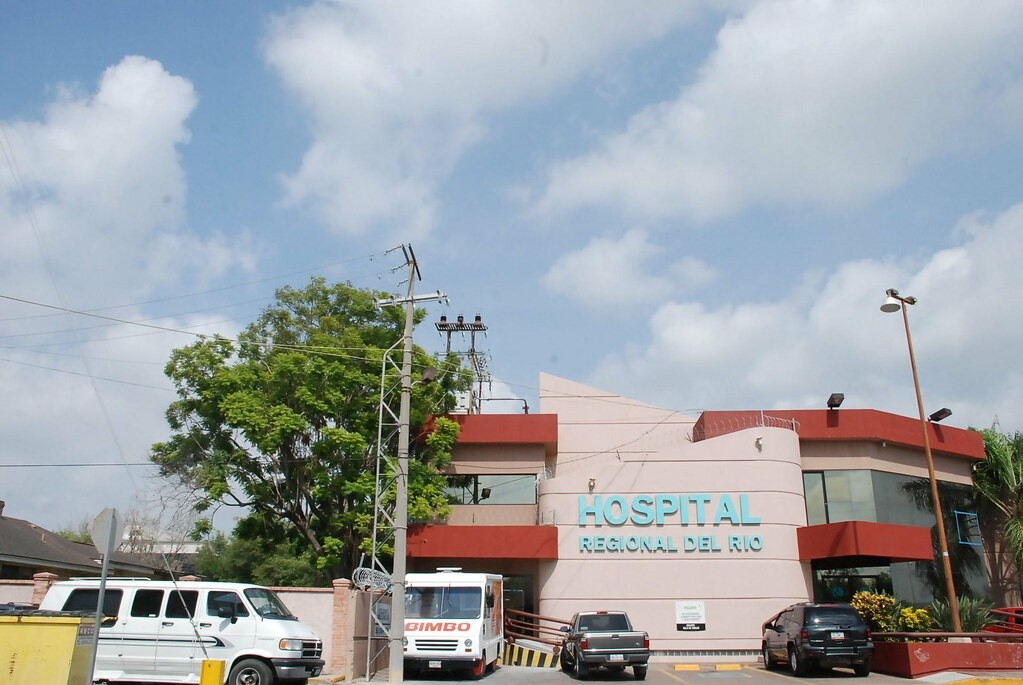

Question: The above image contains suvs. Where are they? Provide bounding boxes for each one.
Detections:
[761,601,874,677]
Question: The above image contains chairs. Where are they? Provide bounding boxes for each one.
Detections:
[583,619,593,624]
[609,617,621,624]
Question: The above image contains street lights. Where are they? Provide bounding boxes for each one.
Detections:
[879,288,966,643]
[371,289,451,684]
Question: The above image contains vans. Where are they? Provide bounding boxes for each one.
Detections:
[37,576,326,685]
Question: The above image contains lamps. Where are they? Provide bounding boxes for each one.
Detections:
[928,407,952,422]
[827,393,845,409]
[757,436,763,444]
[588,478,595,489]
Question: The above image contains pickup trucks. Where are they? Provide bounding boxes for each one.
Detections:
[560,610,650,681]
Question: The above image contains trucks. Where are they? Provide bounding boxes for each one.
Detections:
[401,567,504,677]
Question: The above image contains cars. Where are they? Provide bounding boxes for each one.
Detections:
[980,606,1023,634]
